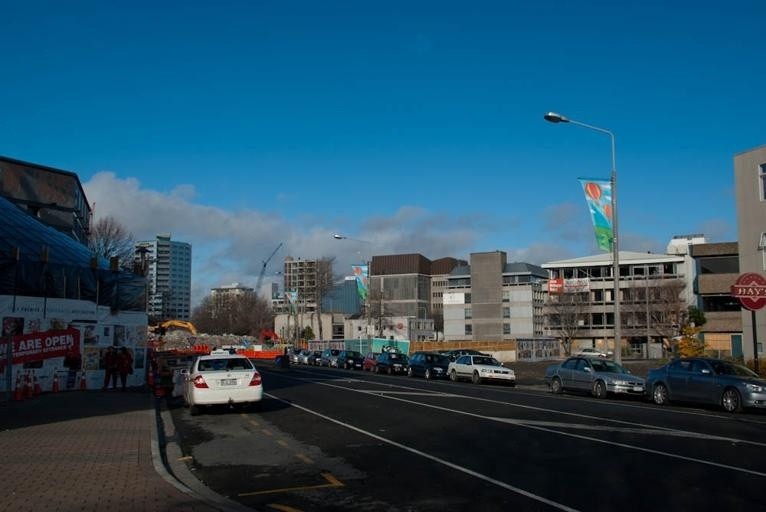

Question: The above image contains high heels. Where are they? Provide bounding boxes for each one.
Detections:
[147,365,154,387]
[51,367,60,393]
[78,368,88,390]
[13,367,42,402]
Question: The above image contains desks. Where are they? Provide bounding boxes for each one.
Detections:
[334,234,372,340]
[543,111,624,368]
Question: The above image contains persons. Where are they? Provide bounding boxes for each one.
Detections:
[115,347,133,388]
[100,346,117,391]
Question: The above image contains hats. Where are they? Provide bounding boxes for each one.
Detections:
[643,355,765,417]
[181,353,264,417]
[544,356,646,402]
[573,347,607,359]
[286,346,518,385]
